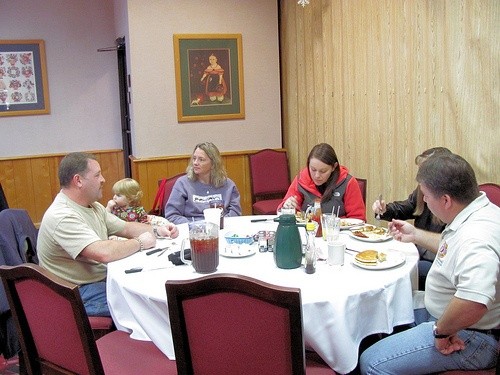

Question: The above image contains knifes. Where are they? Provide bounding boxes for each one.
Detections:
[146,246,169,256]
[251,218,275,222]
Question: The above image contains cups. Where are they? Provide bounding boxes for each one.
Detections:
[281,206,295,215]
[328,241,345,267]
[209,200,225,229]
[320,212,340,241]
[203,208,221,228]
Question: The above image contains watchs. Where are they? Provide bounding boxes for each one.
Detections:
[432,322,449,339]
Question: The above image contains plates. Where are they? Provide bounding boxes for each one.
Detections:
[353,249,406,270]
[296,220,305,226]
[219,244,256,258]
[340,218,393,243]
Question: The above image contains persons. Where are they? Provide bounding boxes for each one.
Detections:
[372,147,452,289]
[37,151,179,316]
[360,153,500,375]
[277,143,367,223]
[165,142,241,225]
[106,178,150,225]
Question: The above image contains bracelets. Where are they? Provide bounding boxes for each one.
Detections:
[153,225,160,236]
[135,238,143,251]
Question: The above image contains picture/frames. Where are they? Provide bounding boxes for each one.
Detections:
[172,34,246,122]
[0,38,52,117]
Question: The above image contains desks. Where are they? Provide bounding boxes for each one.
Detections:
[105,216,419,375]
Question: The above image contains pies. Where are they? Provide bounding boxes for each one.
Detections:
[355,250,379,266]
[365,225,374,231]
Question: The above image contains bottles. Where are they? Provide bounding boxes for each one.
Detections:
[267,230,276,253]
[304,223,316,274]
[257,230,267,253]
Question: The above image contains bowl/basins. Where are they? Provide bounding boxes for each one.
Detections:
[224,230,255,245]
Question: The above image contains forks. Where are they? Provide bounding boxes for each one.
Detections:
[376,193,383,220]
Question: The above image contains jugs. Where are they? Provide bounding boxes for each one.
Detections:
[179,220,219,274]
[306,206,322,237]
[274,213,308,269]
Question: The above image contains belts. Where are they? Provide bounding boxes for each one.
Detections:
[464,327,500,336]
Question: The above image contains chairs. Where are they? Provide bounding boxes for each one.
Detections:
[248,148,291,216]
[0,263,178,375]
[163,274,336,375]
[0,210,112,375]
